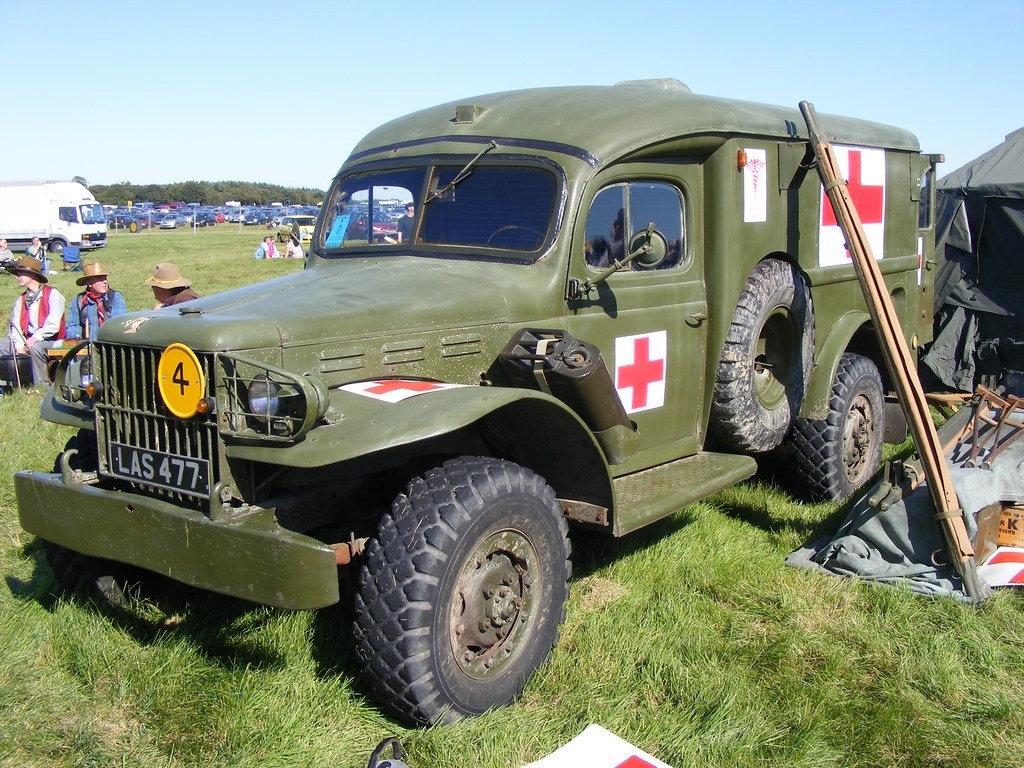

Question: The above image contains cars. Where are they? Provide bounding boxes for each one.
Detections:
[90,201,413,241]
[276,215,330,245]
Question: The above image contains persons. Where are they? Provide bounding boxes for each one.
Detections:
[0,202,416,388]
[582,211,681,273]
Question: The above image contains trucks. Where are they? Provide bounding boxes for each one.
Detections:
[1,180,108,255]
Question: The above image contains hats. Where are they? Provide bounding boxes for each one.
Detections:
[142,263,193,289]
[75,262,112,286]
[6,256,49,284]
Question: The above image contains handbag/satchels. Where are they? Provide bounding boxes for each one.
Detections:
[254,246,265,259]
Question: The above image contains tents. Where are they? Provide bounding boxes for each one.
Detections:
[918,127,1024,397]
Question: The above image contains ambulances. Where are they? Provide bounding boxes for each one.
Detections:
[12,76,947,728]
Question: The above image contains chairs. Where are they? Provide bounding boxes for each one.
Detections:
[60,246,88,272]
[26,247,50,274]
